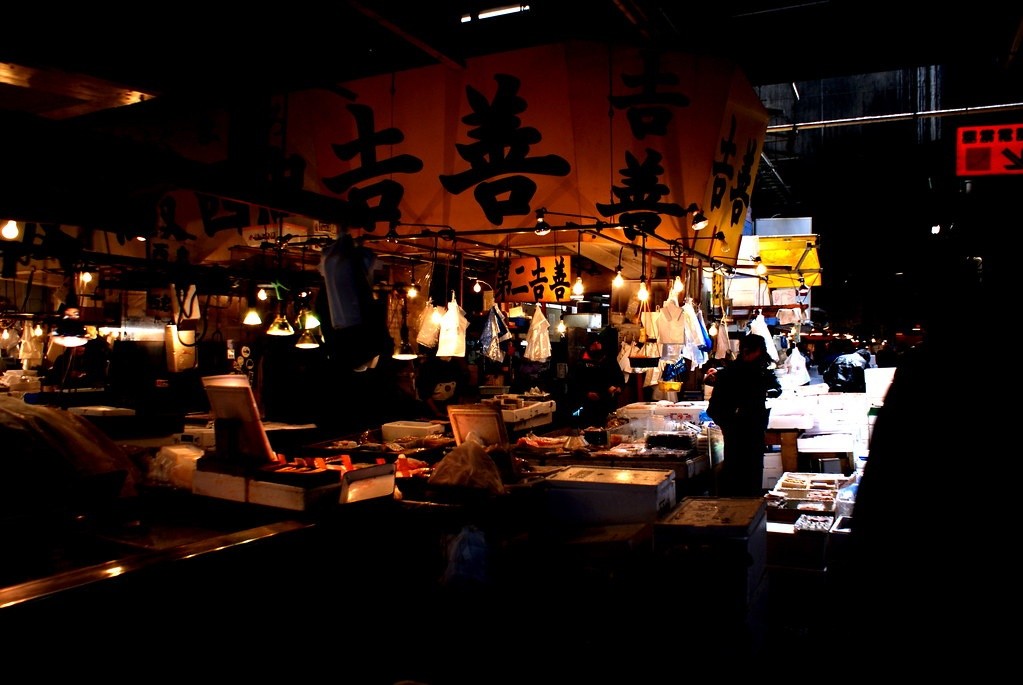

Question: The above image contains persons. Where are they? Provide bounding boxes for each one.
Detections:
[822,340,909,392]
[577,339,626,428]
[0,296,587,436]
[852,175,1023,684]
[706,333,781,497]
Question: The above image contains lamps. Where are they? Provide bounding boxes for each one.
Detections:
[536,209,551,236]
[392,305,418,360]
[385,222,399,243]
[688,203,709,230]
[50,318,89,348]
[244,288,322,349]
[753,256,767,275]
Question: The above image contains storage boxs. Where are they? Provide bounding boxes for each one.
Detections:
[762,367,897,489]
[546,464,677,525]
[192,461,396,511]
[615,399,709,421]
[381,420,444,440]
